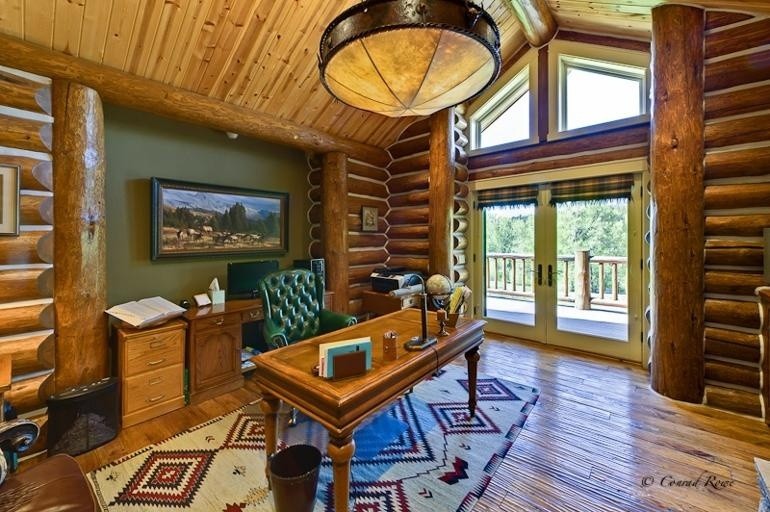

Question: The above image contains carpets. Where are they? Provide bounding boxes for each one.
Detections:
[86,363,541,511]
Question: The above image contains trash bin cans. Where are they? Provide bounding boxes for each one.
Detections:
[268,444,322,512]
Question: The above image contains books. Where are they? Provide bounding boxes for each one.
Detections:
[317,335,373,380]
[104,296,186,329]
[240,346,263,369]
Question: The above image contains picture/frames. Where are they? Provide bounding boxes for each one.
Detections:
[0,164,20,236]
[361,205,379,232]
[150,176,289,262]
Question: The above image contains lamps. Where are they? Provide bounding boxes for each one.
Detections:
[390,274,438,352]
[317,0,501,118]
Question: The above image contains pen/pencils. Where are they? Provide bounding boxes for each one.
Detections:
[384,330,399,339]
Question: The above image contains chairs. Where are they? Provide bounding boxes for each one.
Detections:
[258,268,357,351]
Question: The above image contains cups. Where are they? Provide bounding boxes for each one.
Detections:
[382,336,398,361]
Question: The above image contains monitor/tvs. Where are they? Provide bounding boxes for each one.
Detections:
[226,259,280,302]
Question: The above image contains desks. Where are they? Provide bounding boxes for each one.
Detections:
[250,307,489,512]
[362,290,421,321]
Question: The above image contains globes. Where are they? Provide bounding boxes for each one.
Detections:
[426,273,454,336]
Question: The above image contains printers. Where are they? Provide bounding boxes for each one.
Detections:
[370,270,424,292]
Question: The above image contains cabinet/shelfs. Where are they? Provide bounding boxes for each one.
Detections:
[112,318,189,430]
[182,298,264,407]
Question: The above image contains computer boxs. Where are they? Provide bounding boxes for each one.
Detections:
[293,258,326,294]
